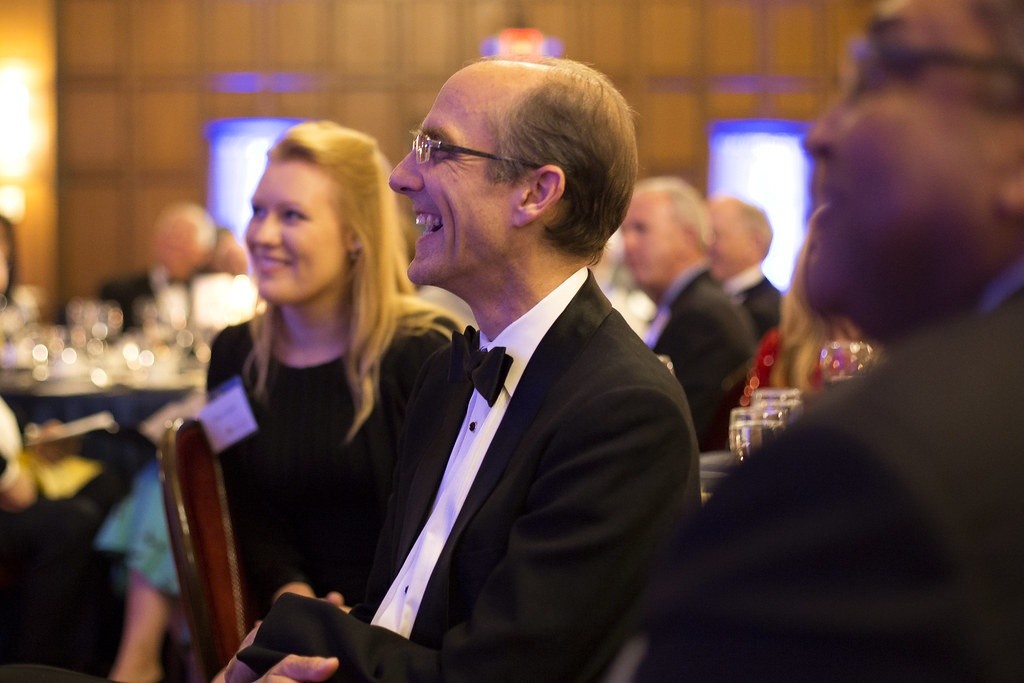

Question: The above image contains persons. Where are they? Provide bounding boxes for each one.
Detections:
[112,123,460,682]
[620,177,782,454]
[101,206,214,335]
[618,1,1022,683]
[0,398,80,513]
[94,465,183,683]
[217,61,697,682]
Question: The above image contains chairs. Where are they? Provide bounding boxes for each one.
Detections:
[156,417,256,683]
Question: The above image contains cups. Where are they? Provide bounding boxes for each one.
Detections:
[728,387,804,470]
[820,340,873,384]
[0,271,259,386]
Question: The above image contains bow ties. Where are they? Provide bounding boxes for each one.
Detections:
[449,325,508,407]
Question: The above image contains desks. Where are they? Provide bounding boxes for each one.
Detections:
[0,370,199,485]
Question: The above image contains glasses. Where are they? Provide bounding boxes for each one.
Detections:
[411,130,571,202]
[850,33,1024,95]
